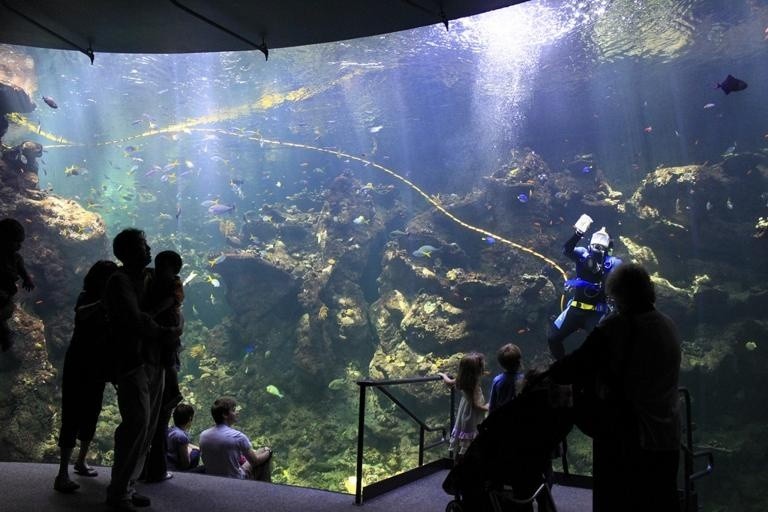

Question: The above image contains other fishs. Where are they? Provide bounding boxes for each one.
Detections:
[0,112,350,360]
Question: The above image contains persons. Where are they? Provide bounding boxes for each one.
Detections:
[199,398,273,482]
[529,265,681,511]
[166,404,206,473]
[0,217,184,511]
[548,213,623,360]
[2,218,33,353]
[450,343,525,463]
[52,228,182,512]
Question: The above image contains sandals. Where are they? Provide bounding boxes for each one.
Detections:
[74,461,98,477]
[56,476,79,490]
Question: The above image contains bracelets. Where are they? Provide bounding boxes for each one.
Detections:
[264,449,273,458]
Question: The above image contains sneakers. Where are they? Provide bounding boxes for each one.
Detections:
[106,491,150,512]
